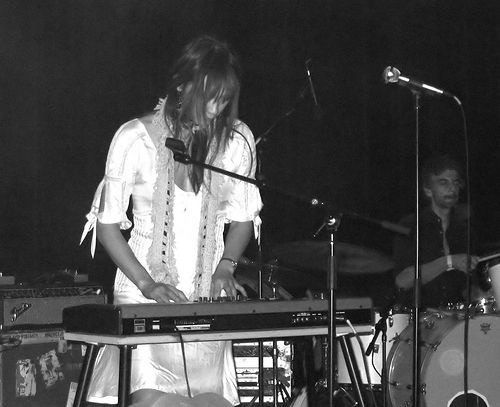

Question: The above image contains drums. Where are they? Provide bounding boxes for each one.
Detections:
[382,305,499,407]
[461,251,500,315]
[310,305,413,388]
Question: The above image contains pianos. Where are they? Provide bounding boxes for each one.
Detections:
[61,296,374,347]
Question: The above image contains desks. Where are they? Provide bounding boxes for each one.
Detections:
[63,324,374,407]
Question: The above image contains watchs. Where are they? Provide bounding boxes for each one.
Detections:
[446,255,454,272]
[219,258,238,268]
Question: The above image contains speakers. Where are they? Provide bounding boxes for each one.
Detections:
[0,328,86,407]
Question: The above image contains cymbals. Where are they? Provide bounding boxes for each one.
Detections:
[265,238,398,276]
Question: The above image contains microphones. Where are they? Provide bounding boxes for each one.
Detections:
[307,69,317,105]
[383,66,454,99]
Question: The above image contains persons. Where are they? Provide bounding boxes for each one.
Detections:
[392,157,485,311]
[79,37,263,407]
